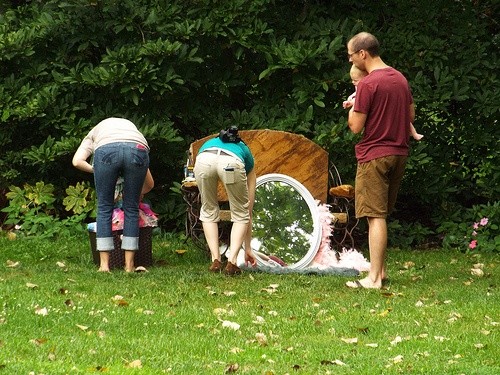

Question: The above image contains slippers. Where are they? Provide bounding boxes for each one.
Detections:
[346,280,363,289]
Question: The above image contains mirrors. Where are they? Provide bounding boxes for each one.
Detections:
[245,173,323,267]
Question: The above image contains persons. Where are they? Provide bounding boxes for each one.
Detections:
[345,32,415,291]
[342,64,424,140]
[71,117,154,272]
[194,136,257,273]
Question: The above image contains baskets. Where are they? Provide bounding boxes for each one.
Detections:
[88,226,153,267]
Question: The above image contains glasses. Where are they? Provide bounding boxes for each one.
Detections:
[348,48,366,59]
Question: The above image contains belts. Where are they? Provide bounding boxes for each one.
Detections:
[203,150,233,157]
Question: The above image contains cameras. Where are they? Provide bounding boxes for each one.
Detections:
[219,125,242,143]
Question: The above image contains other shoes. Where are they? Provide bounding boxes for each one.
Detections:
[225,262,241,274]
[210,260,226,272]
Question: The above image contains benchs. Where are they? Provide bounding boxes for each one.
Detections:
[181,124,360,252]
